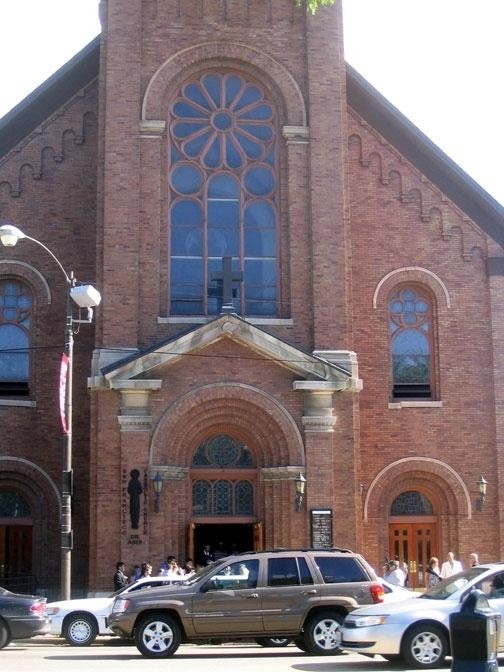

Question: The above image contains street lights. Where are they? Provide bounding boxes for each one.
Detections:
[0,222,76,601]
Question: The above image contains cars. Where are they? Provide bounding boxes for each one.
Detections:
[47,575,292,648]
[0,586,52,648]
[337,560,504,669]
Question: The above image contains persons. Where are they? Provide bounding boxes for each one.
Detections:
[114,542,257,592]
[488,578,504,598]
[381,560,408,588]
[440,551,464,593]
[423,556,442,590]
[469,553,482,567]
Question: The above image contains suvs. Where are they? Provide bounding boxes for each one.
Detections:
[109,547,384,658]
